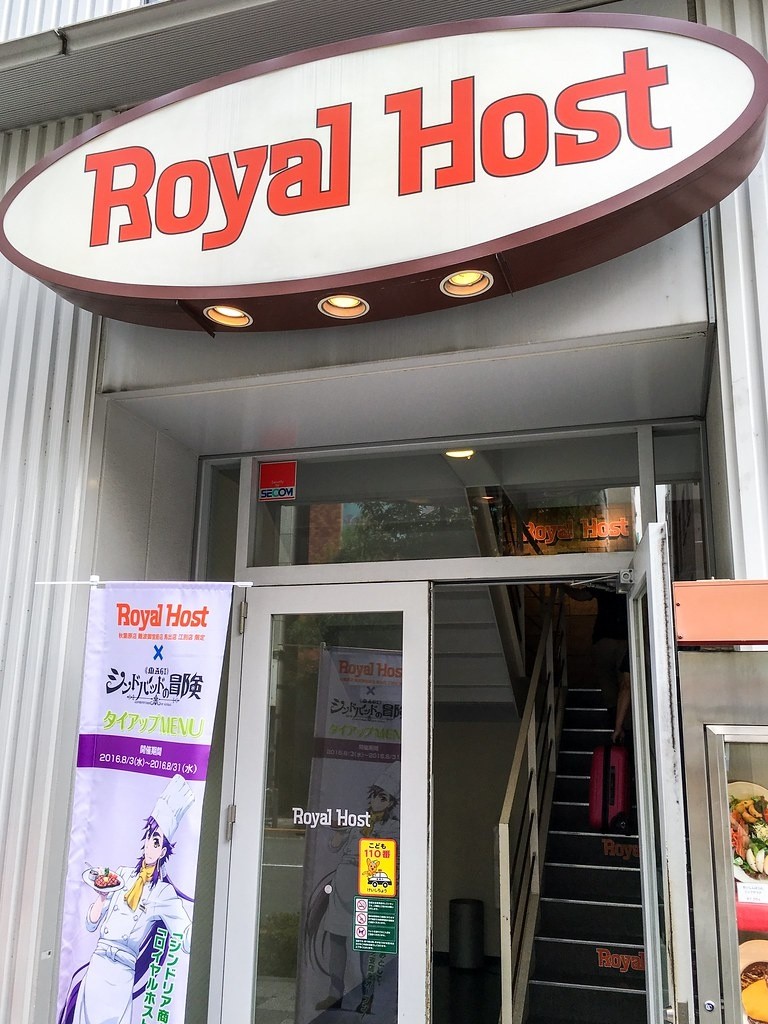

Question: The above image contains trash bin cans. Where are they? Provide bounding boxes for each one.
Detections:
[449,898,484,970]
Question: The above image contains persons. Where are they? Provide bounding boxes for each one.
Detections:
[550,582,637,836]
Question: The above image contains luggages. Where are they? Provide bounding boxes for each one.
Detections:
[589,735,631,834]
